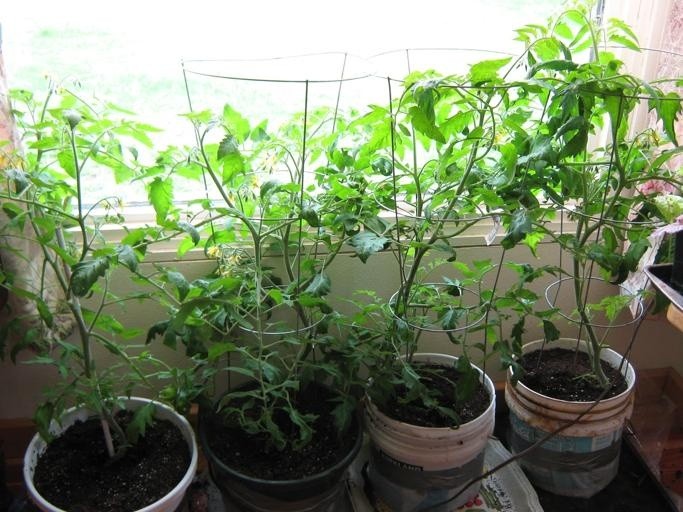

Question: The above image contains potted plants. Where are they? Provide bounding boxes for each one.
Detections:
[491,2,683,501]
[167,67,369,512]
[2,73,204,512]
[353,55,534,511]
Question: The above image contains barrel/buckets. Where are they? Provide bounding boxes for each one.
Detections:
[363,352,496,512]
[24,396,201,512]
[505,338,636,499]
[198,376,363,512]
